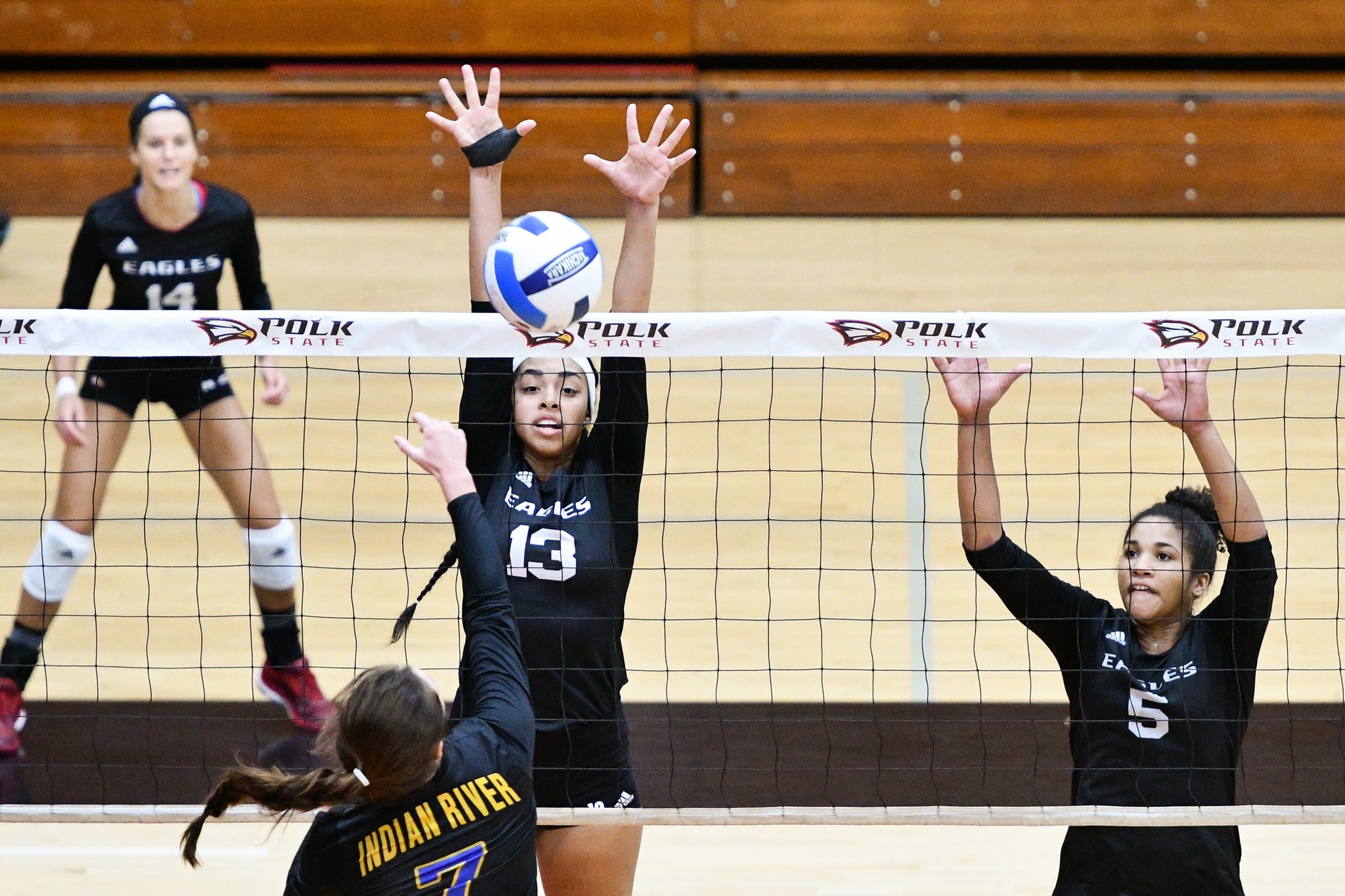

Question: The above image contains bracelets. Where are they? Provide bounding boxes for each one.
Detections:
[55,375,78,398]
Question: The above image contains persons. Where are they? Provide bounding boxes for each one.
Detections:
[391,61,695,896]
[0,86,334,754]
[932,359,1278,896]
[175,413,539,896]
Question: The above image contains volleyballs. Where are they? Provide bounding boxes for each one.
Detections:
[483,210,604,334]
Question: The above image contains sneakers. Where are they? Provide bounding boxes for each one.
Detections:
[0,677,27,755]
[254,656,329,731]
